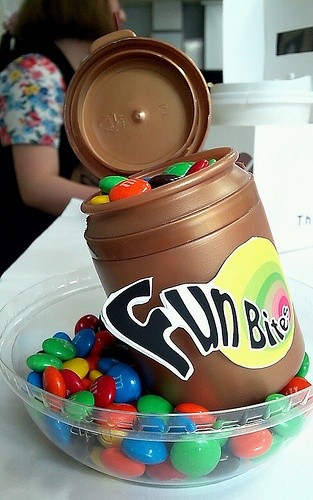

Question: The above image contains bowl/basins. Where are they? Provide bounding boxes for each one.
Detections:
[0,266,313,487]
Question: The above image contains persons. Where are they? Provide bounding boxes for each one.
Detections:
[0,0,127,277]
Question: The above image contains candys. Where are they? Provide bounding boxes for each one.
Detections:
[85,158,219,207]
[25,314,313,481]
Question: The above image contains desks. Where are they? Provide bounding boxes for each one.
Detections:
[0,193,313,500]
